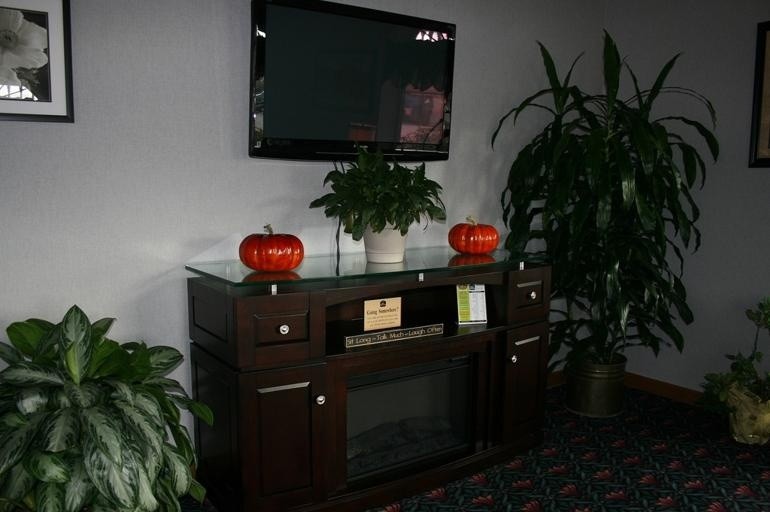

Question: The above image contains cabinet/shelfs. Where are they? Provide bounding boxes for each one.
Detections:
[182,237,564,509]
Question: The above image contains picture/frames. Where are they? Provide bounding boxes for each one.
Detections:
[1,0,77,124]
[746,19,769,171]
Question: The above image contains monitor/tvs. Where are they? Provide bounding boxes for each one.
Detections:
[249,0,456,162]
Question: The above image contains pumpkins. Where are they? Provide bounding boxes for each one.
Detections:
[448,224,499,254]
[239,234,304,272]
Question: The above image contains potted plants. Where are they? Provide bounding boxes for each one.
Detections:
[486,24,723,425]
[304,134,449,277]
[695,293,769,449]
